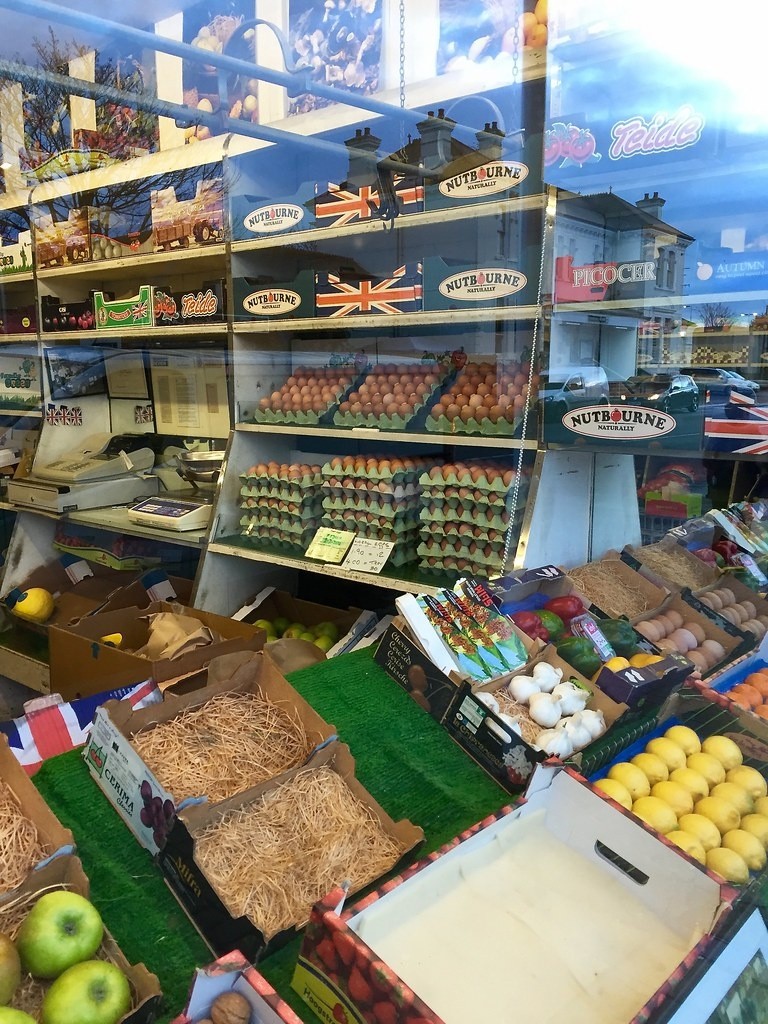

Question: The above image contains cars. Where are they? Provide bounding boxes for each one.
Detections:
[621,373,698,415]
[727,370,759,392]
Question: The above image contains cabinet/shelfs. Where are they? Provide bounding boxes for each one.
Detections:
[0,38,768,621]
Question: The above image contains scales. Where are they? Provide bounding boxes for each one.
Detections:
[127,450,226,533]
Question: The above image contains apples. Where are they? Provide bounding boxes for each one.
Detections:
[0,891,130,1024]
[253,617,341,653]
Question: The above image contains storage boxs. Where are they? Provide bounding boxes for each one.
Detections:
[0,521,201,635]
[0,651,426,1024]
[232,586,396,659]
[232,244,542,323]
[48,601,267,702]
[290,755,749,1024]
[0,426,39,480]
[0,179,225,274]
[232,132,543,242]
[638,491,703,546]
[0,278,227,334]
[0,345,43,411]
[373,498,767,909]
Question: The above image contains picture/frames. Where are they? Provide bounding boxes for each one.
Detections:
[43,345,108,400]
[648,904,768,1024]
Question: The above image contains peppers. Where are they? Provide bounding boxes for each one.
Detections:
[690,541,740,570]
[514,595,662,683]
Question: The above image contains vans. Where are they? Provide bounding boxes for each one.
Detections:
[679,366,757,401]
[531,366,610,416]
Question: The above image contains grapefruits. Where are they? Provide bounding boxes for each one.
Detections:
[15,588,54,621]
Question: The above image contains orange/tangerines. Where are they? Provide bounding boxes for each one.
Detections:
[722,667,768,722]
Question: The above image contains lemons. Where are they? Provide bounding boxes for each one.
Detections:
[592,725,768,884]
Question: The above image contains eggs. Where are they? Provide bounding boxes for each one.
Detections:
[634,587,768,673]
[238,363,543,577]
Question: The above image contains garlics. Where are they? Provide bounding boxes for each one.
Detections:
[474,663,605,758]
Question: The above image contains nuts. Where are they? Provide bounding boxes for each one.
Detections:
[195,992,252,1024]
